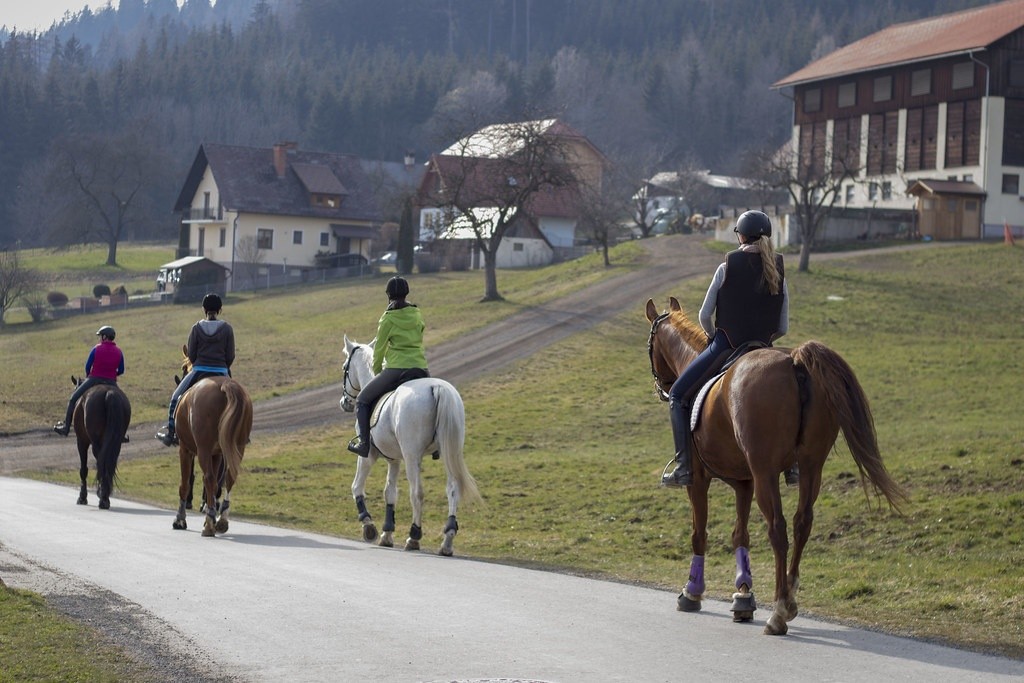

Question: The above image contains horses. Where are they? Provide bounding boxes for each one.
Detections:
[173,344,253,537]
[71,374,131,510]
[339,333,488,556]
[645,296,912,636]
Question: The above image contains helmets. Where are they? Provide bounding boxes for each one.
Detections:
[737,210,771,236]
[385,276,409,298]
[96,326,115,338]
[202,294,222,311]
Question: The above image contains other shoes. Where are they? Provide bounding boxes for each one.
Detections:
[155,432,173,446]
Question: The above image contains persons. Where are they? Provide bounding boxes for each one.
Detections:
[663,210,799,487]
[54,326,129,443]
[157,293,250,447]
[347,275,440,460]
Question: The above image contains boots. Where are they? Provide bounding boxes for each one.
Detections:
[662,397,692,484]
[348,400,373,458]
[784,464,799,486]
[54,403,75,436]
